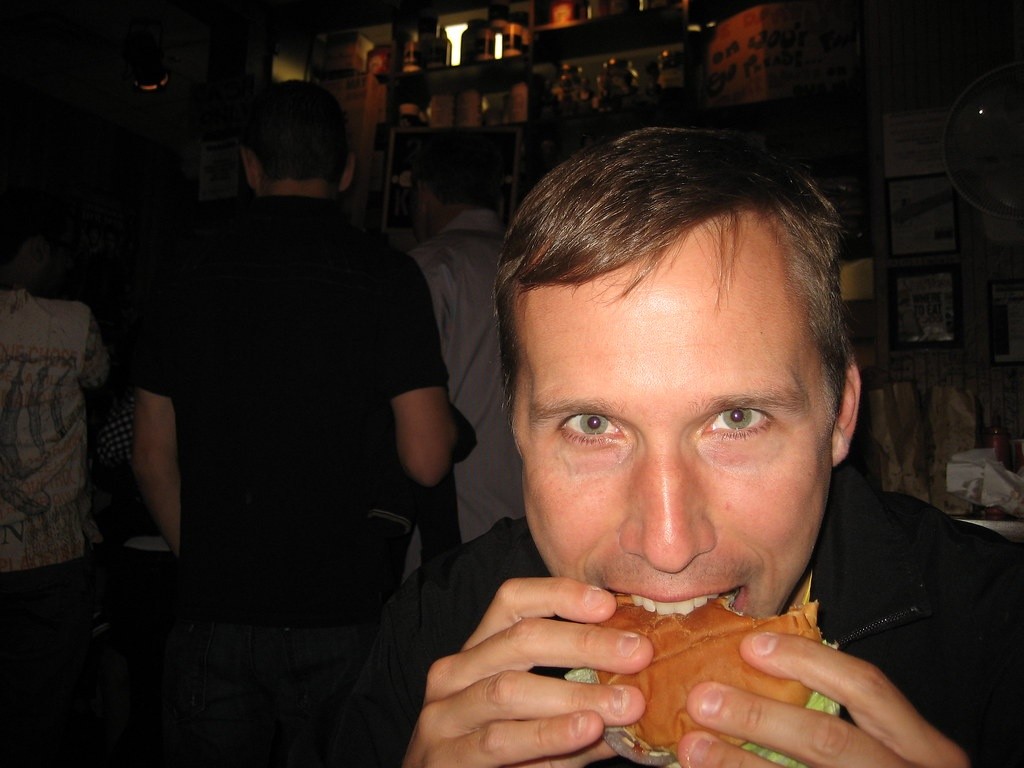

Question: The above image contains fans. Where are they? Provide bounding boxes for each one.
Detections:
[940,61,1024,222]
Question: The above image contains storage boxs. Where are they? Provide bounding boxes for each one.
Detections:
[325,32,376,56]
[322,54,368,73]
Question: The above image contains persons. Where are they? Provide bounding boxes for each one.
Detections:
[285,127,1024,768]
[0,83,525,768]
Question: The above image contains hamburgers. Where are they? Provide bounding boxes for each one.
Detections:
[562,591,841,768]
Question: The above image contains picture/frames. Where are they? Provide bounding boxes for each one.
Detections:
[883,171,961,260]
[988,278,1024,367]
[886,262,964,351]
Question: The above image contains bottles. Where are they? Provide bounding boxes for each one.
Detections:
[982,427,1010,521]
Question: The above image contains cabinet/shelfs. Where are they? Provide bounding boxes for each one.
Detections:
[302,1,892,369]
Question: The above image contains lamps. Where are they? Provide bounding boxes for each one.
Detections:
[120,16,172,94]
